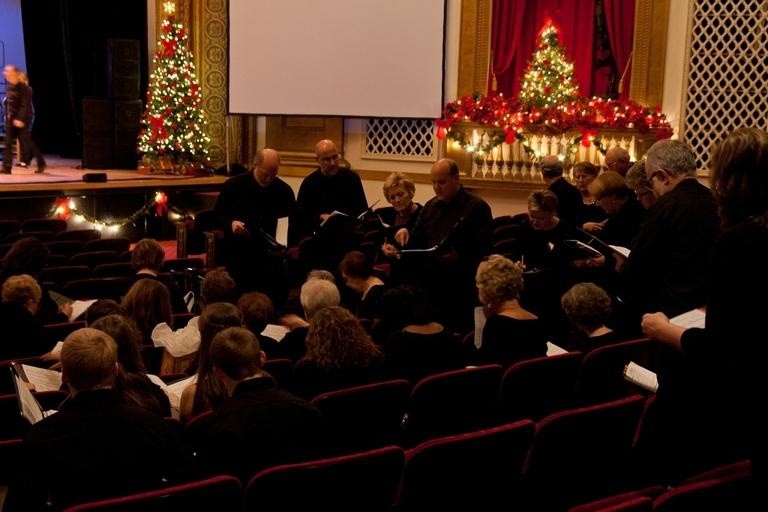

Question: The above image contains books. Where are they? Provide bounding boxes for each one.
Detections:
[12,324,291,425]
[321,198,383,226]
[396,216,463,261]
[579,229,629,259]
[275,212,290,250]
[69,295,99,325]
[543,340,576,358]
[474,302,500,350]
[621,358,658,394]
[548,220,602,258]
[666,303,706,331]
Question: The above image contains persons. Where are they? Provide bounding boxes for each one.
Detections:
[517,132,767,486]
[2,241,272,386]
[14,72,37,167]
[214,141,542,398]
[1,65,46,175]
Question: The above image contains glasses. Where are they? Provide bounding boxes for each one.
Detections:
[634,189,649,200]
[642,173,658,191]
